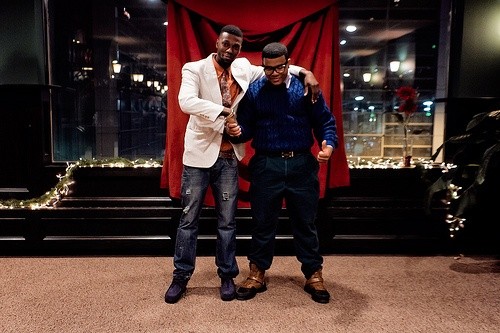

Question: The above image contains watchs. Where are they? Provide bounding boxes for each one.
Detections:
[226,108,235,119]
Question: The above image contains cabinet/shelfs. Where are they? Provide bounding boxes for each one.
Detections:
[339,112,441,160]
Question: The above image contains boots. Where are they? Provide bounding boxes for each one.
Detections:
[236,262,268,301]
[304,265,330,303]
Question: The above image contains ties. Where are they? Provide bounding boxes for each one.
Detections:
[221,69,232,108]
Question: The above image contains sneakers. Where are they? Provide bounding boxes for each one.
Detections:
[219,274,237,301]
[164,275,189,304]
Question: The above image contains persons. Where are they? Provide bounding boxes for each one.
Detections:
[226,43,339,304]
[165,24,319,303]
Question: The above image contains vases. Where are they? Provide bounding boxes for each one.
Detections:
[401,138,415,167]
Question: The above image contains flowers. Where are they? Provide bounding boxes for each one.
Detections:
[383,85,422,155]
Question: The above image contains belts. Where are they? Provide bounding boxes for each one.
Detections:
[279,151,295,158]
[218,151,235,159]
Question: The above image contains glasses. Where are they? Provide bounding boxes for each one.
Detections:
[263,59,288,76]
